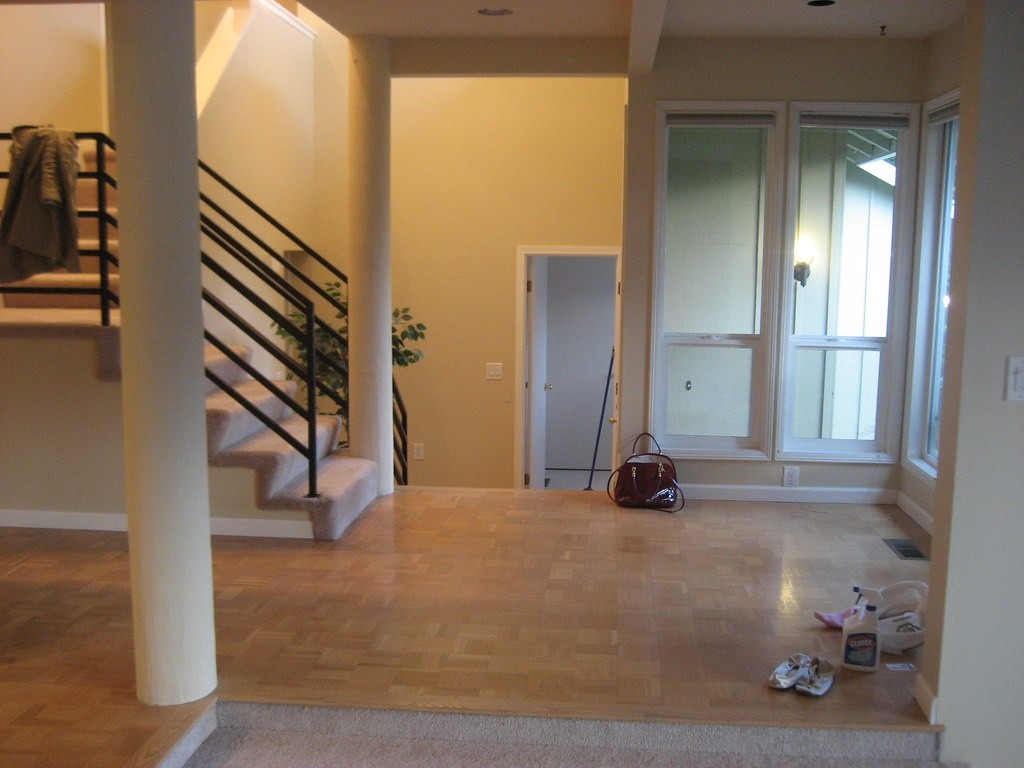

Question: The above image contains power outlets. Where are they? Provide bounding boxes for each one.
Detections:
[783,466,799,487]
[413,443,424,460]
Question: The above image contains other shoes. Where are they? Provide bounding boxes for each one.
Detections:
[769,654,811,689]
[796,657,834,696]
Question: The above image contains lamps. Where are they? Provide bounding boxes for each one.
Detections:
[794,261,811,287]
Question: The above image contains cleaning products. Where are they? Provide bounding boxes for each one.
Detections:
[840,586,883,674]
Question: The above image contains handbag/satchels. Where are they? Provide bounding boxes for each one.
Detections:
[607,433,685,513]
